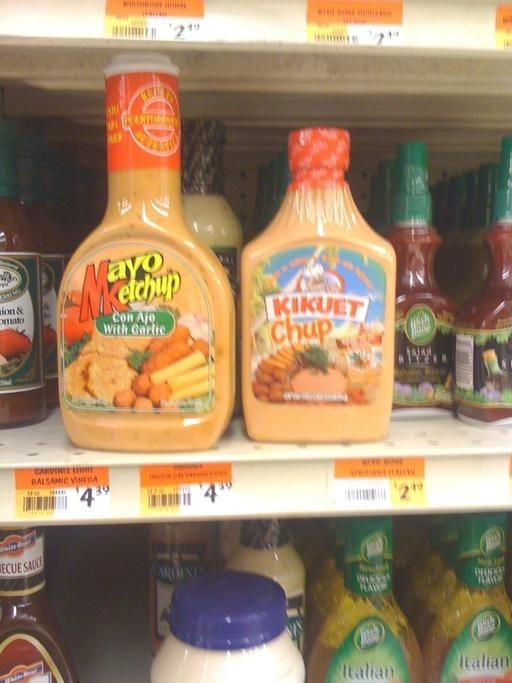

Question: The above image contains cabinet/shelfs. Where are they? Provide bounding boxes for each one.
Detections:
[0,1,512,683]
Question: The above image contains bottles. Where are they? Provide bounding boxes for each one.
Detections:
[0,527,77,682]
[0,48,511,453]
[148,510,512,683]
